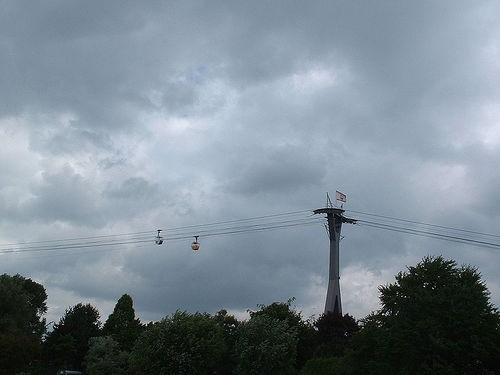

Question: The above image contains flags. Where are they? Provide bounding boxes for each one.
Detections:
[336,190,346,203]
